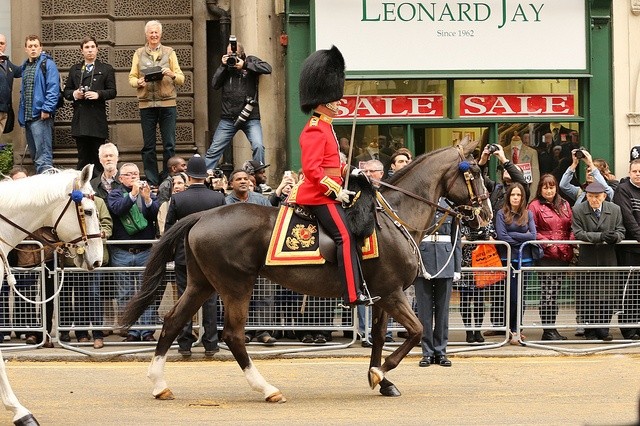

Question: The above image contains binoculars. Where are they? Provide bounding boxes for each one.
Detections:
[78,85,89,100]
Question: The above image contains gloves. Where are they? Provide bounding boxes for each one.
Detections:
[351,169,364,176]
[453,272,461,283]
[335,189,356,204]
[602,233,618,245]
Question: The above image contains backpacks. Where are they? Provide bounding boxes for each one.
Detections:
[40,57,64,109]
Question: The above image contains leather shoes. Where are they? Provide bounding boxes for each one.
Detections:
[142,334,156,341]
[257,333,278,343]
[205,347,220,357]
[123,335,141,342]
[349,294,381,308]
[541,329,563,340]
[26,336,37,344]
[43,340,54,348]
[60,334,71,341]
[555,329,568,340]
[435,355,452,367]
[94,339,104,348]
[361,341,371,346]
[178,349,192,356]
[244,334,251,342]
[598,332,613,340]
[78,337,89,342]
[419,356,434,367]
[626,332,639,340]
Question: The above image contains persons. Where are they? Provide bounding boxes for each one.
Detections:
[246,160,271,346]
[128,19,185,184]
[478,142,531,335]
[64,36,118,182]
[11,170,50,347]
[558,147,614,345]
[390,152,410,173]
[417,198,461,367]
[202,35,272,175]
[223,168,272,343]
[540,122,571,148]
[356,159,396,346]
[460,224,494,345]
[163,156,226,355]
[526,174,574,341]
[1,34,30,131]
[249,176,256,191]
[614,141,640,340]
[268,169,297,344]
[397,147,415,163]
[18,33,60,171]
[106,162,162,344]
[503,136,541,203]
[298,169,330,345]
[294,46,381,314]
[37,233,71,348]
[353,142,391,181]
[574,182,613,343]
[497,183,536,346]
[159,156,187,205]
[157,171,186,235]
[91,142,126,204]
[77,192,111,350]
[209,168,227,190]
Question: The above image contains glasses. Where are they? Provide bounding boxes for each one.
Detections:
[364,170,383,173]
[120,171,139,177]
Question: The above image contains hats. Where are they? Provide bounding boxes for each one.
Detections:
[243,160,271,176]
[585,181,605,193]
[629,146,640,162]
[300,44,346,115]
[185,157,209,179]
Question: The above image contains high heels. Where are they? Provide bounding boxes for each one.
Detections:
[574,328,585,336]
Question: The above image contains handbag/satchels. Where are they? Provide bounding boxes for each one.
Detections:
[140,65,165,83]
[530,242,545,262]
[120,187,149,235]
[472,239,507,288]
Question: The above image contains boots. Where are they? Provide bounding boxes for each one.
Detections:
[466,331,475,343]
[474,326,484,342]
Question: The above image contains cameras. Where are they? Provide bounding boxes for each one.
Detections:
[487,144,500,155]
[78,84,91,97]
[211,167,224,178]
[576,146,590,159]
[226,34,239,69]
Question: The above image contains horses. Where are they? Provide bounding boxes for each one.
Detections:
[115,137,493,404]
[0,161,103,426]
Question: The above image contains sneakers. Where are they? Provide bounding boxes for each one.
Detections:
[301,335,313,343]
[313,335,327,343]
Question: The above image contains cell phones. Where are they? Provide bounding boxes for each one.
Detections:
[136,180,148,189]
[284,169,293,181]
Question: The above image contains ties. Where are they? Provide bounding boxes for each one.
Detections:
[594,209,601,218]
[512,146,519,164]
[86,64,93,71]
